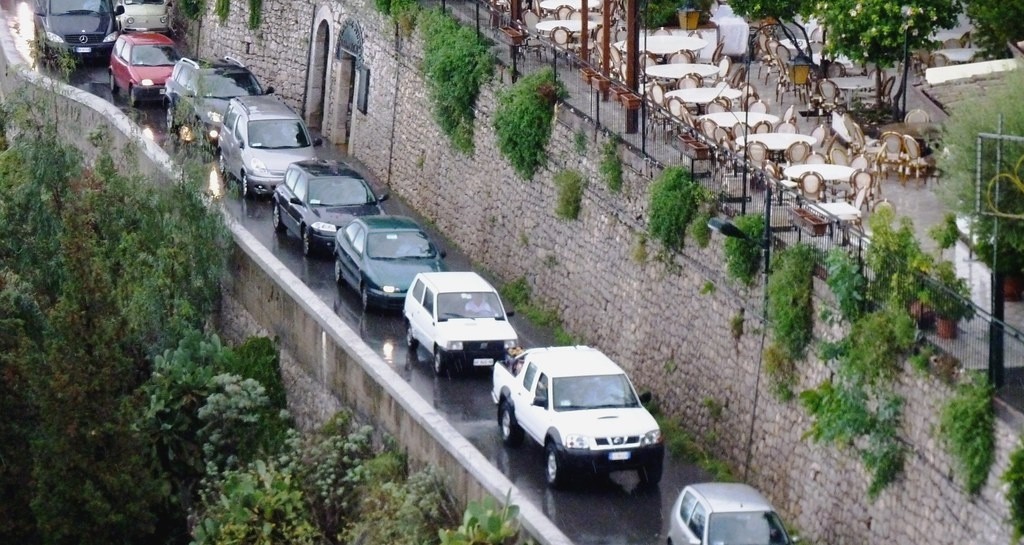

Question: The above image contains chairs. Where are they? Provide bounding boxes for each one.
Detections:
[488,0,990,234]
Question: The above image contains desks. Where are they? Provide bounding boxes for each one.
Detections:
[797,202,861,247]
[924,33,960,43]
[613,36,707,63]
[880,122,945,172]
[932,48,994,65]
[535,20,597,43]
[664,88,742,115]
[700,112,780,140]
[645,63,720,90]
[806,53,852,93]
[735,133,817,164]
[828,77,876,111]
[769,37,815,61]
[540,0,600,13]
[783,164,856,203]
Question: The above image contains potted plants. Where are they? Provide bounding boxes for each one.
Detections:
[975,237,1024,301]
[925,278,976,339]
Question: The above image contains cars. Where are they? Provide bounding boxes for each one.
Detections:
[113,0,173,36]
[107,30,184,107]
[332,213,448,314]
[665,480,800,544]
[271,158,390,258]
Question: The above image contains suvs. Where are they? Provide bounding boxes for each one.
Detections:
[161,55,275,156]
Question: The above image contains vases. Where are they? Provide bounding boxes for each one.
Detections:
[609,87,629,101]
[506,31,524,45]
[579,67,597,82]
[678,134,696,152]
[688,142,709,159]
[591,76,611,90]
[498,27,515,40]
[620,93,642,108]
[792,209,814,226]
[803,216,828,235]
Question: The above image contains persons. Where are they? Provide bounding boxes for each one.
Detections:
[465,293,491,313]
[584,380,624,405]
[349,186,366,203]
[395,235,424,255]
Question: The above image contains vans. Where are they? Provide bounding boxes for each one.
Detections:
[402,271,520,378]
[32,0,125,67]
[215,93,323,203]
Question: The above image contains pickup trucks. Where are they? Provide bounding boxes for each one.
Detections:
[490,341,666,487]
